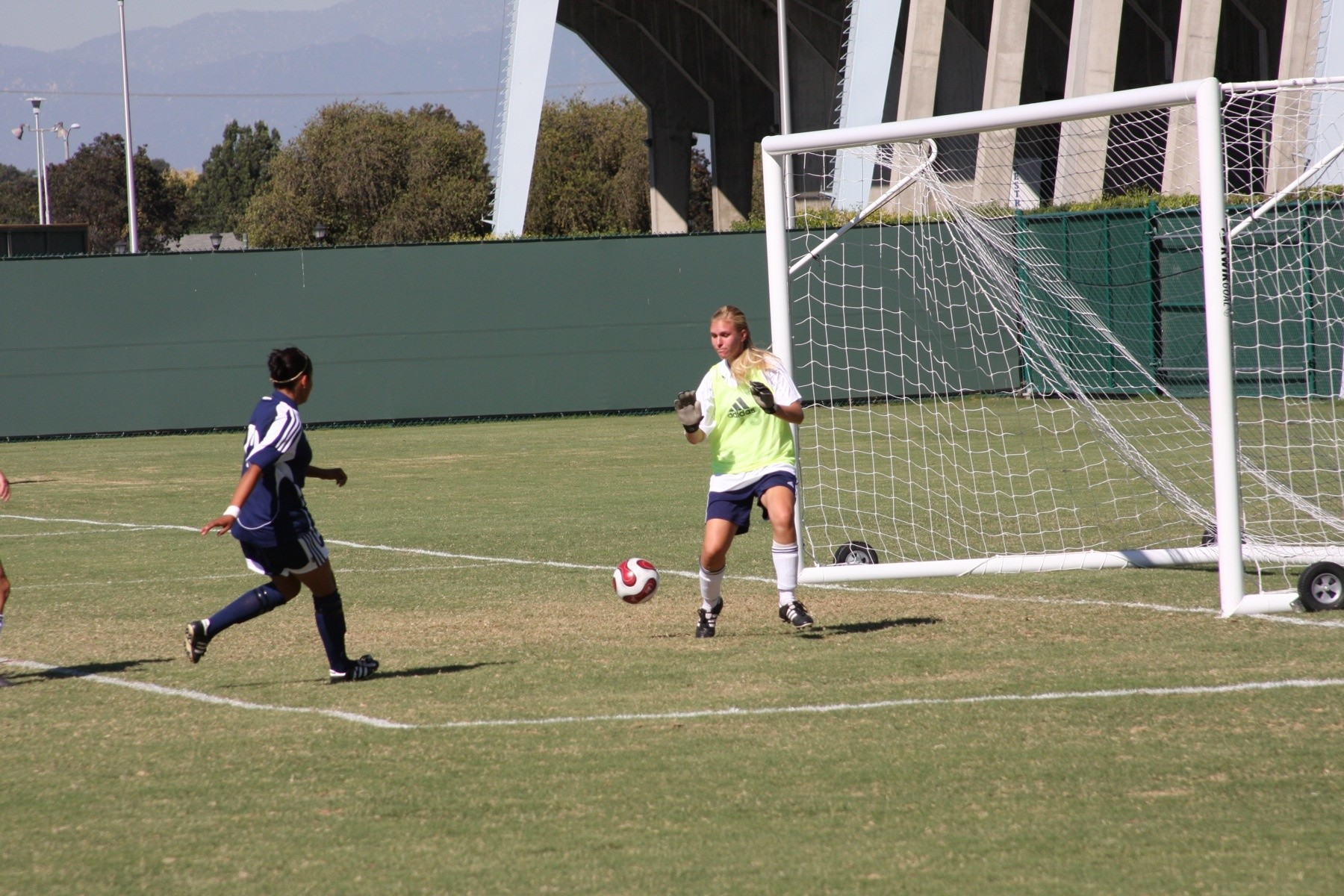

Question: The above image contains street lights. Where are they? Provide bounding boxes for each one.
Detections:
[24,95,47,225]
[65,123,81,163]
[11,120,69,225]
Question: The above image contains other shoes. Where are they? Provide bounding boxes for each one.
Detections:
[0,679,14,687]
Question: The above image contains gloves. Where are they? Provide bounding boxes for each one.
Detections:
[749,380,777,414]
[674,389,704,434]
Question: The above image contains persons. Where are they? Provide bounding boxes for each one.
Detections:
[673,305,814,638]
[0,471,11,630]
[184,346,380,684]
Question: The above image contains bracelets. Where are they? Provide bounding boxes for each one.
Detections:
[223,506,241,518]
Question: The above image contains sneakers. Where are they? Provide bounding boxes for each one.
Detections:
[695,597,723,638]
[329,654,379,684]
[779,600,813,629]
[185,618,210,664]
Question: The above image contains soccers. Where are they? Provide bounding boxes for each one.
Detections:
[611,558,660,605]
